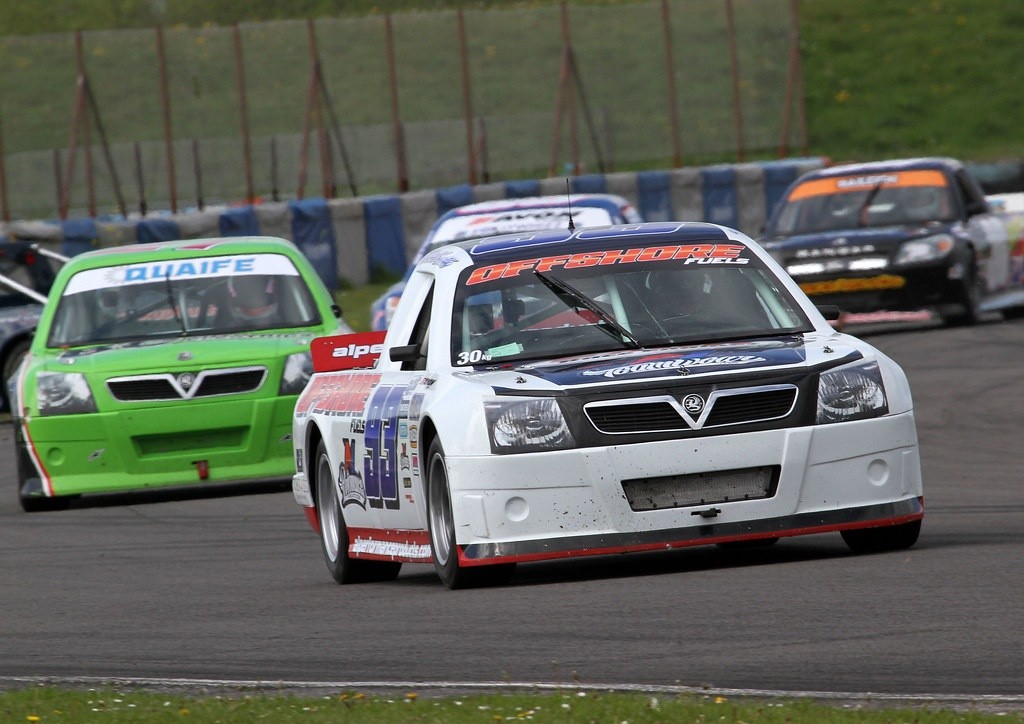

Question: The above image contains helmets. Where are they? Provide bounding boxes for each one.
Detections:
[899,187,939,220]
[226,275,279,326]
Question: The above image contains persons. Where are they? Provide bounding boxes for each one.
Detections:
[630,268,760,335]
[897,186,941,220]
[213,274,284,329]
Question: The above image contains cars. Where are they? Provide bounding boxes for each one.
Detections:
[292,177,924,592]
[0,236,359,511]
[763,156,1023,327]
